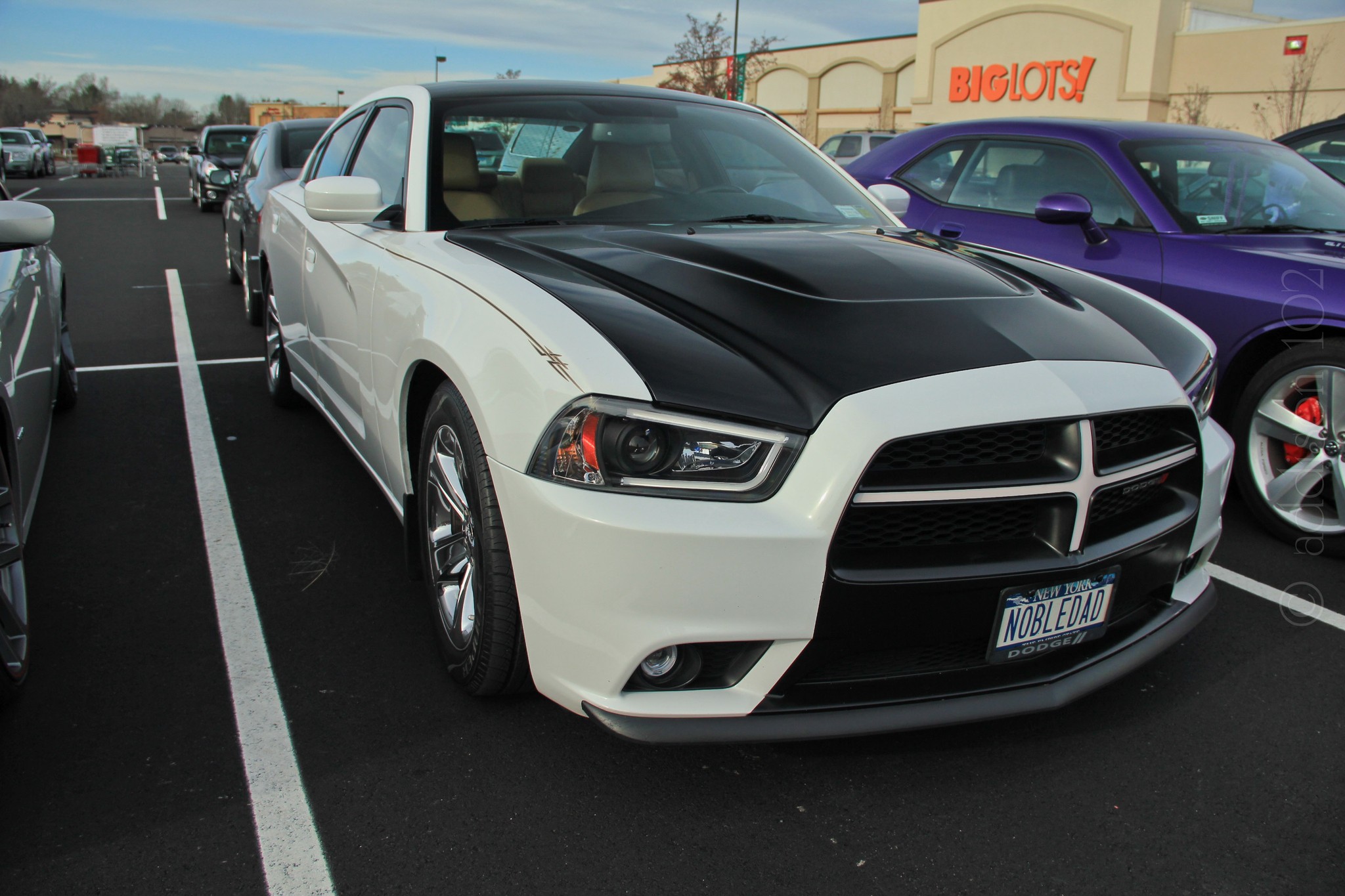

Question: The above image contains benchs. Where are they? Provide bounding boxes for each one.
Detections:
[479,158,589,223]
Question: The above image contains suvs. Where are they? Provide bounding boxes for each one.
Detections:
[817,130,953,171]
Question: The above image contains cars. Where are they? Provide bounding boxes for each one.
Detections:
[1,127,56,176]
[754,116,1345,545]
[187,124,261,214]
[0,129,45,175]
[156,146,181,164]
[1180,117,1345,229]
[0,178,80,699]
[222,118,338,327]
[259,82,1236,748]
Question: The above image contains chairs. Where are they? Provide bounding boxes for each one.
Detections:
[209,139,230,153]
[1101,167,1157,228]
[574,145,664,218]
[442,132,510,222]
[989,164,1051,215]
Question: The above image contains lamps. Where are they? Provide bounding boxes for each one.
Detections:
[1284,35,1308,54]
